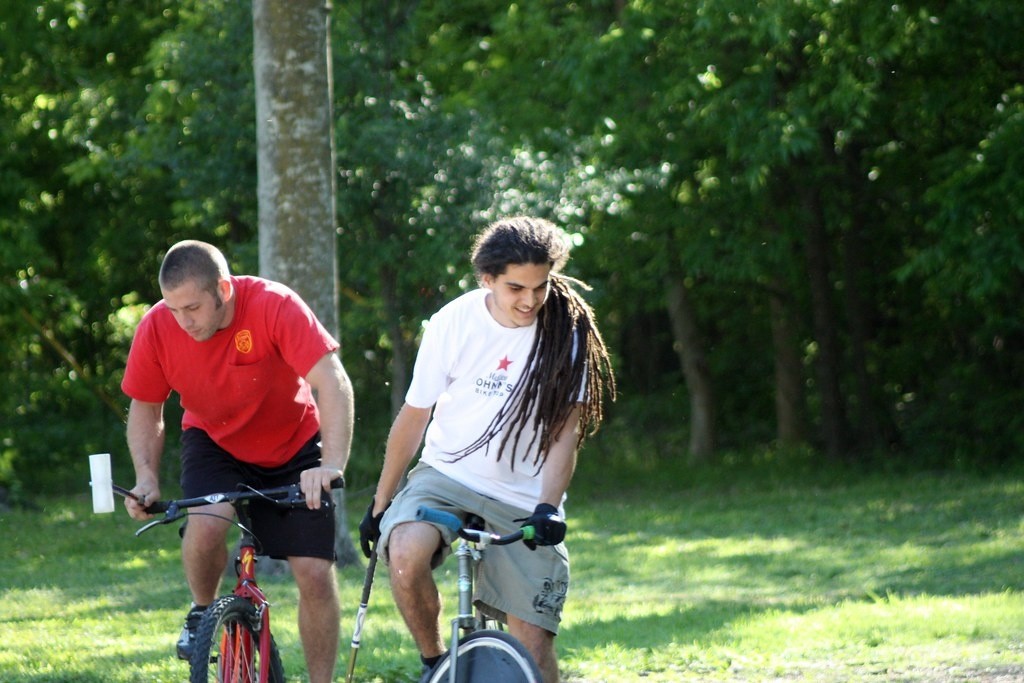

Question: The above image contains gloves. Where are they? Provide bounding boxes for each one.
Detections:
[513,503,567,550]
[359,500,392,558]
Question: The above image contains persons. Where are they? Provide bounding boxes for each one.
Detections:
[121,241,353,682]
[359,219,618,683]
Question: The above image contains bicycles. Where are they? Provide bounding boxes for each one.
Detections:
[414,504,558,683]
[133,473,345,683]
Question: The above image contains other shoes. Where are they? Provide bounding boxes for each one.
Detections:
[177,602,207,659]
